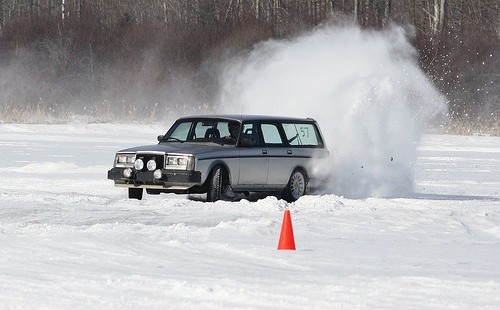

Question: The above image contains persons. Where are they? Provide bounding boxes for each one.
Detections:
[221,121,255,146]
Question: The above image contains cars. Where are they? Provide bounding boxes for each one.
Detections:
[107,112,331,204]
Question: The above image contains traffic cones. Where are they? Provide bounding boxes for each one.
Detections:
[277,210,295,251]
